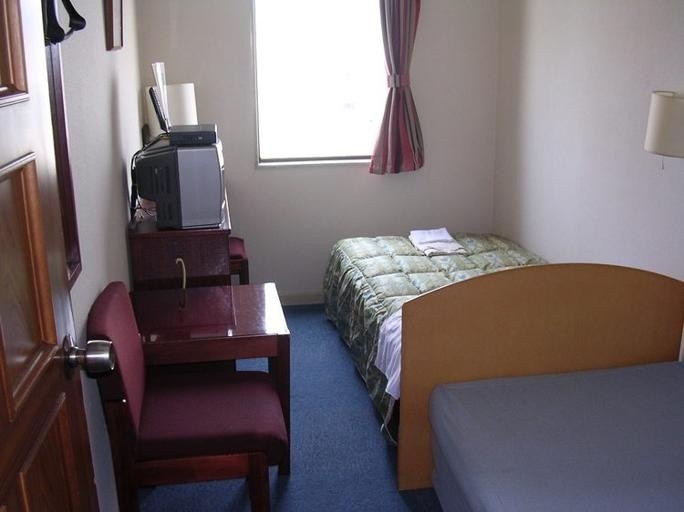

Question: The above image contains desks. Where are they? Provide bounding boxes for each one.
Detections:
[128,186,232,290]
[128,282,290,438]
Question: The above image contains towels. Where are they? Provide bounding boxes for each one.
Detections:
[410,227,467,255]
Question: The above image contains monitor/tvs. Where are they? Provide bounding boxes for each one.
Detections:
[135,133,227,230]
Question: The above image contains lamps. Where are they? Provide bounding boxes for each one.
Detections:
[642,90,684,170]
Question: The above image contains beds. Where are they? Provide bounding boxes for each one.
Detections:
[322,231,550,447]
[427,360,684,512]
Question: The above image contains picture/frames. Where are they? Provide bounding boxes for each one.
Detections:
[104,0,123,51]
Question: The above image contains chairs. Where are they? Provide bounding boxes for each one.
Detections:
[86,282,290,512]
[228,236,249,285]
